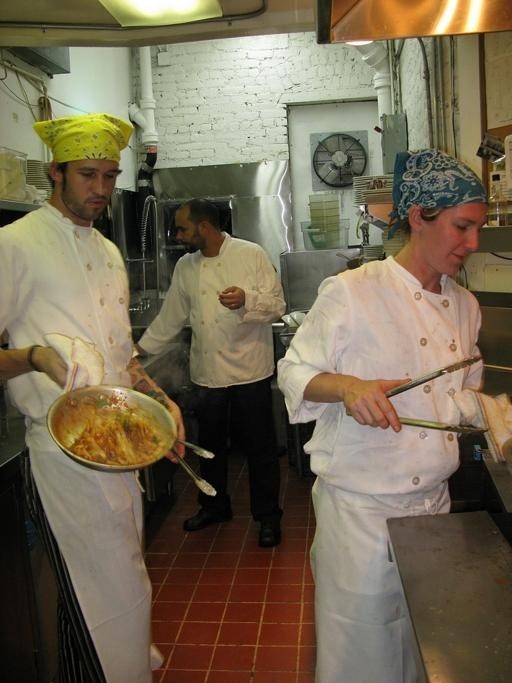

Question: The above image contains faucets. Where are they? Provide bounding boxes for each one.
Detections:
[140,195,158,260]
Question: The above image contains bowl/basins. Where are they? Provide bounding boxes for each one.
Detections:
[282,310,306,329]
[299,218,349,251]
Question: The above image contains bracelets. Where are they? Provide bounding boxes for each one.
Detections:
[26,344,40,376]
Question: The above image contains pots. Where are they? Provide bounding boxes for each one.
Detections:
[45,384,177,472]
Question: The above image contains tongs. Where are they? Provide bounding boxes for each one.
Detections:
[169,437,217,497]
[346,354,490,435]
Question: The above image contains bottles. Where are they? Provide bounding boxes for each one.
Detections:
[486,173,509,227]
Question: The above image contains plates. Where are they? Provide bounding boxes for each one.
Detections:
[24,158,53,203]
[352,174,397,206]
[362,244,383,261]
[380,224,408,260]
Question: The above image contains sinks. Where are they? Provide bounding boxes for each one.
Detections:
[131,327,156,361]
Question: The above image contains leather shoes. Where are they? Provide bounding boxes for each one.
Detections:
[258,521,281,547]
[182,506,229,531]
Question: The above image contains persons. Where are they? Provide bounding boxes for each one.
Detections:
[276,146,489,680]
[1,113,185,682]
[137,197,287,548]
